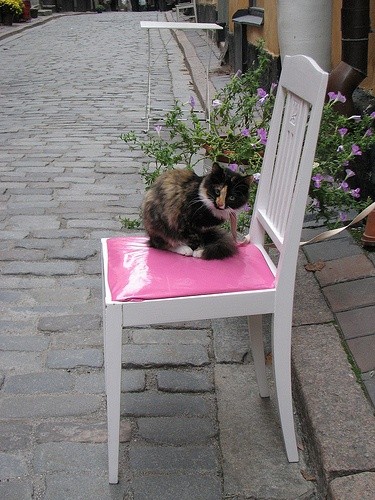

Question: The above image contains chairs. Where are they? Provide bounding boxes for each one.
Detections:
[176,0,198,23]
[101,55,329,484]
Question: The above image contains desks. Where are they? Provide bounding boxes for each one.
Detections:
[140,20,223,132]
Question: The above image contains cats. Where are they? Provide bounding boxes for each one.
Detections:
[142,161,254,260]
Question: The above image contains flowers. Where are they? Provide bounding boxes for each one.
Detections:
[111,37,375,244]
[0,0,26,21]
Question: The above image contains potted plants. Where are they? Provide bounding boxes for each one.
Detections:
[95,5,105,14]
